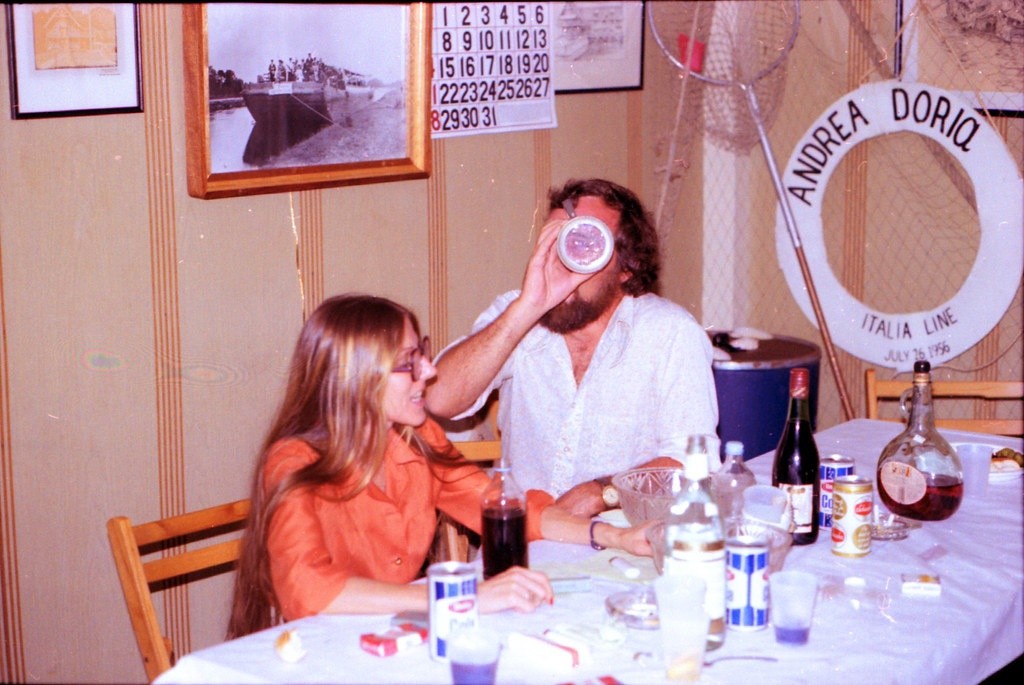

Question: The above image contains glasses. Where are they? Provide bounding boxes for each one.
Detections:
[389,336,432,381]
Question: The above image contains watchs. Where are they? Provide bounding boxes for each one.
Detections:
[594,478,620,510]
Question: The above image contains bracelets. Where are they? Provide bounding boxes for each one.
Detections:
[589,520,605,550]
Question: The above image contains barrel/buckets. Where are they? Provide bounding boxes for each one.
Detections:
[710,327,821,463]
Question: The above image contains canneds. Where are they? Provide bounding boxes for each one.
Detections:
[426,561,478,663]
[722,535,771,631]
[831,475,873,557]
[819,453,855,532]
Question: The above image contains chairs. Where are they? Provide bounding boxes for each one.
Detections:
[109,431,501,685]
[863,369,1024,437]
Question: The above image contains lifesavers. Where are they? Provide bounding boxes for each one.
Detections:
[775,80,1024,372]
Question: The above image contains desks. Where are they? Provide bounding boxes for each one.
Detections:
[152,418,1023,684]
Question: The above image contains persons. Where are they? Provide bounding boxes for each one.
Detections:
[268,53,319,82]
[425,178,720,520]
[224,293,653,642]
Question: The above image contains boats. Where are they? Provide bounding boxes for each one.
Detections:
[239,82,373,124]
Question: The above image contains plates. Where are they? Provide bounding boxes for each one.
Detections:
[949,442,1024,483]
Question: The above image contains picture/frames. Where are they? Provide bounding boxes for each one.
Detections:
[5,3,145,120]
[893,0,1023,118]
[547,1,645,95]
[182,1,434,197]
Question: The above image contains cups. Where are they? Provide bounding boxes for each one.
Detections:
[556,199,614,273]
[654,576,709,682]
[741,485,788,524]
[956,445,991,499]
[444,628,501,685]
[768,571,818,644]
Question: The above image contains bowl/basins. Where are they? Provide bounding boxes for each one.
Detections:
[646,518,794,576]
[610,466,748,529]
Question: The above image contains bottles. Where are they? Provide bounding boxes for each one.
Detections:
[711,440,756,518]
[664,436,728,651]
[876,361,964,522]
[480,458,529,581]
[770,368,821,545]
